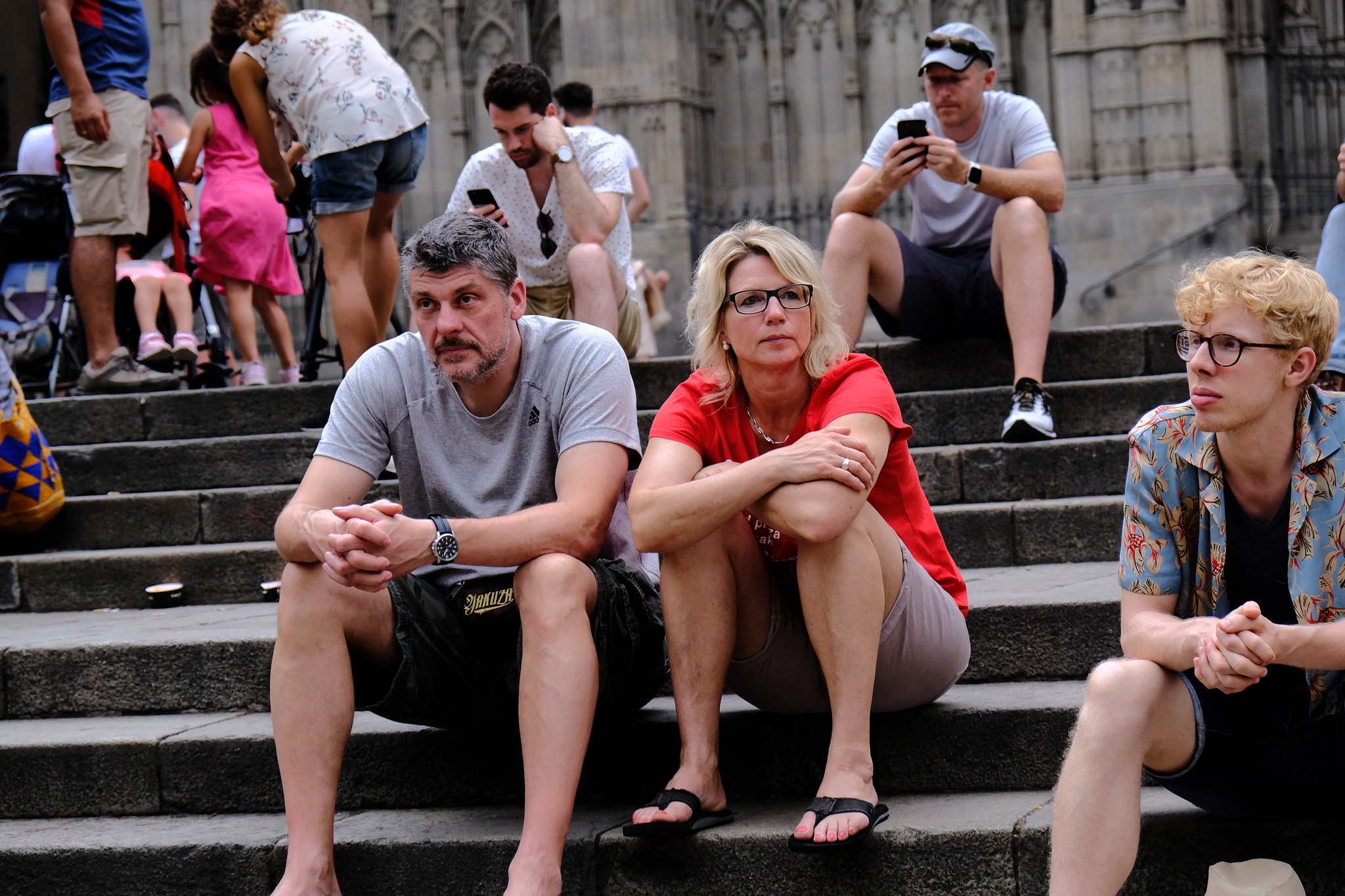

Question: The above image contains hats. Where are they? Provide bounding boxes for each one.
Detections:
[917,21,996,77]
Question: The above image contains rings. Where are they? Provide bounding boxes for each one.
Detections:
[81,130,87,135]
[842,458,850,469]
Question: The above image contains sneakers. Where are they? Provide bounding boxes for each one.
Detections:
[1001,377,1057,439]
[75,346,179,394]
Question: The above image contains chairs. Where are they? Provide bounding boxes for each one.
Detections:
[0,173,403,396]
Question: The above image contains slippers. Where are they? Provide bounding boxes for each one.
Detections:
[787,795,890,852]
[622,787,735,839]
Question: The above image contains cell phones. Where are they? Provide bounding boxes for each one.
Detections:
[468,189,508,228]
[897,118,928,168]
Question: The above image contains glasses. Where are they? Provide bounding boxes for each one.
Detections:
[1169,329,1297,367]
[924,32,993,69]
[721,284,814,315]
[536,210,558,260]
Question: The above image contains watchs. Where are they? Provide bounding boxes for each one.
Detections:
[962,160,983,191]
[426,511,459,565]
[549,145,576,166]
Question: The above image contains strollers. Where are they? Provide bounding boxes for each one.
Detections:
[266,159,410,384]
[1,151,236,400]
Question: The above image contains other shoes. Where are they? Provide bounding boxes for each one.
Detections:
[237,361,268,386]
[136,329,173,361]
[171,330,199,360]
[270,364,304,384]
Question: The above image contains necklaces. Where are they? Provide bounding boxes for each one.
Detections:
[747,406,789,445]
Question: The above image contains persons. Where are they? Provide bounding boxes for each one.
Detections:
[0,0,432,396]
[267,212,669,896]
[623,217,971,852]
[445,62,643,358]
[1316,139,1345,392]
[821,21,1069,442]
[630,261,670,360]
[551,81,651,217]
[1048,244,1345,896]
